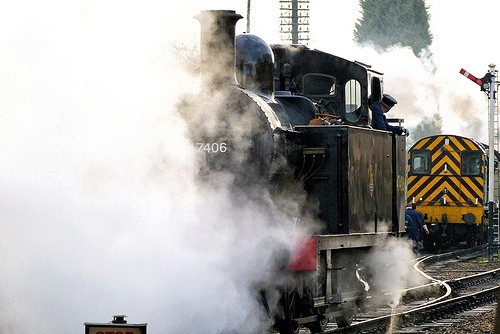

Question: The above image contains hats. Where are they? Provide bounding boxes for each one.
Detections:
[381,94,398,109]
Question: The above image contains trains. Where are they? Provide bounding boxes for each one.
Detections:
[405,135,499,253]
[172,7,411,332]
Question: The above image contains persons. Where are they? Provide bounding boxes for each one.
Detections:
[370,94,410,136]
